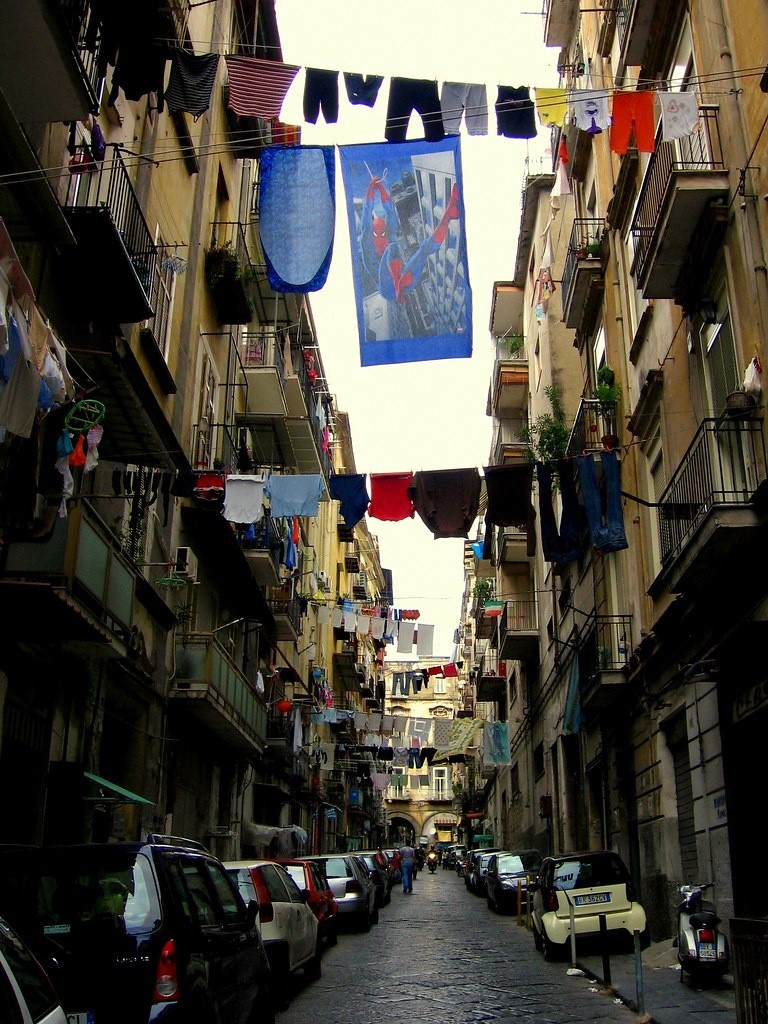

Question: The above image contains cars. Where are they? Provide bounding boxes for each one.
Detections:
[446,844,650,958]
[0,832,402,1024]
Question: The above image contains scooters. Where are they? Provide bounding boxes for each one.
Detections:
[674,881,730,985]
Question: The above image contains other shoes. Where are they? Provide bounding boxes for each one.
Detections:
[408,890,411,893]
[403,889,407,893]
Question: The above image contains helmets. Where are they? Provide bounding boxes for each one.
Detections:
[430,845,435,850]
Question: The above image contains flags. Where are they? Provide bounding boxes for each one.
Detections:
[338,135,473,368]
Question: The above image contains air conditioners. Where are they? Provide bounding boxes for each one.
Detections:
[174,546,201,584]
[318,570,332,593]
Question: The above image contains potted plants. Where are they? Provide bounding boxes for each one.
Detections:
[591,361,623,450]
[726,385,758,418]
[505,331,524,359]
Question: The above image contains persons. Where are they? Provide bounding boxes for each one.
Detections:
[442,847,449,871]
[437,846,443,866]
[426,845,437,856]
[413,845,419,880]
[417,846,426,871]
[398,838,415,892]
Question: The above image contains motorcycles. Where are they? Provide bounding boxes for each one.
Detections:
[425,848,441,874]
